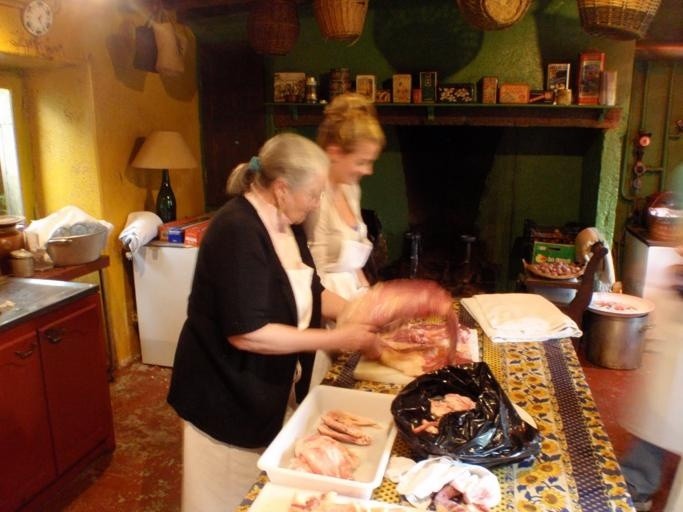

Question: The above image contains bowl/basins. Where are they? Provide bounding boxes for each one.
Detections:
[47,224,106,267]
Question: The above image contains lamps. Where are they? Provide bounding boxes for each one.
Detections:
[131,129,198,221]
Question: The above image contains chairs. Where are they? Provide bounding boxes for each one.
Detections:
[523,241,608,355]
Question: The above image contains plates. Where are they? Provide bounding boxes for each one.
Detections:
[587,292,654,318]
[407,466,500,510]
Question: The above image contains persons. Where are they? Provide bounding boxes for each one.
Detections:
[387,224,479,299]
[166,134,385,512]
[299,93,386,294]
[360,209,387,286]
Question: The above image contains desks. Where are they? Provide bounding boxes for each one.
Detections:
[30,255,119,384]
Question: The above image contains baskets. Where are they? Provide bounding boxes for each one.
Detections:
[312,0,369,48]
[647,190,683,245]
[457,0,533,33]
[247,0,302,58]
[576,0,662,43]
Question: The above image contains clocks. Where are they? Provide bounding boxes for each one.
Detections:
[22,1,54,39]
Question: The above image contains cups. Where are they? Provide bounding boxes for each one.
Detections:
[598,70,617,105]
[9,249,34,278]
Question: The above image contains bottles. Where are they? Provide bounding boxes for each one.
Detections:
[305,76,318,103]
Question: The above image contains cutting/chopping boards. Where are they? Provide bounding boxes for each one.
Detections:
[353,328,478,381]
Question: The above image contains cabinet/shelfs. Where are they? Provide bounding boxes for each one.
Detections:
[0,276,115,508]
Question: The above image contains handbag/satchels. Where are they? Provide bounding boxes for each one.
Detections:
[151,17,199,79]
[132,24,158,75]
